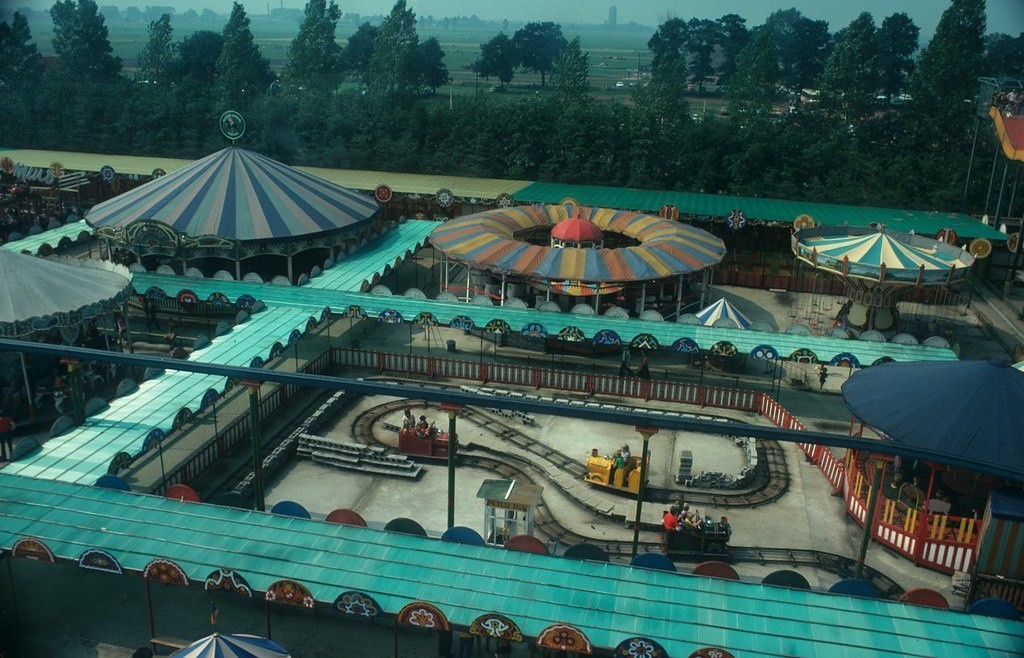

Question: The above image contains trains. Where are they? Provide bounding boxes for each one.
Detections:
[398,426,460,462]
[658,510,733,561]
[583,448,652,494]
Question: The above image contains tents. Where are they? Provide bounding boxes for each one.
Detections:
[428,205,726,297]
[841,360,1024,479]
[84,146,381,259]
[695,299,752,329]
[791,219,979,295]
[0,246,134,343]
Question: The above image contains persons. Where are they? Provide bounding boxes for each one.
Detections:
[403,407,428,439]
[165,319,178,344]
[145,298,164,335]
[0,412,17,463]
[112,308,131,344]
[661,499,728,533]
[610,444,630,484]
[912,476,920,489]
